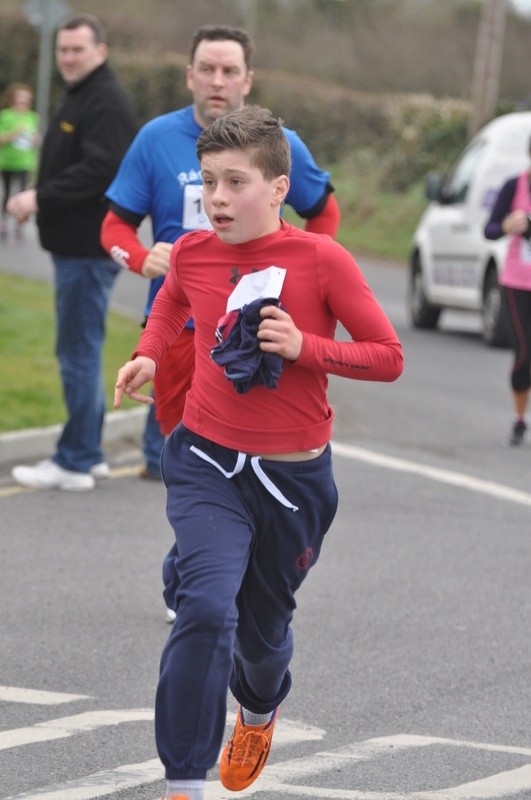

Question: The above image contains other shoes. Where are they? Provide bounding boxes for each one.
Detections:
[93,460,109,477]
[165,608,177,625]
[511,421,527,445]
[10,459,95,492]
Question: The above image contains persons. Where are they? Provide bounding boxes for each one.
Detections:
[139,388,165,481]
[99,23,340,625]
[483,130,531,445]
[6,11,140,492]
[0,80,45,239]
[113,104,403,800]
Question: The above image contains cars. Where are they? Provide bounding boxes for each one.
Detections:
[405,109,530,352]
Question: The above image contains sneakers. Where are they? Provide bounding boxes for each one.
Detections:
[220,703,279,791]
